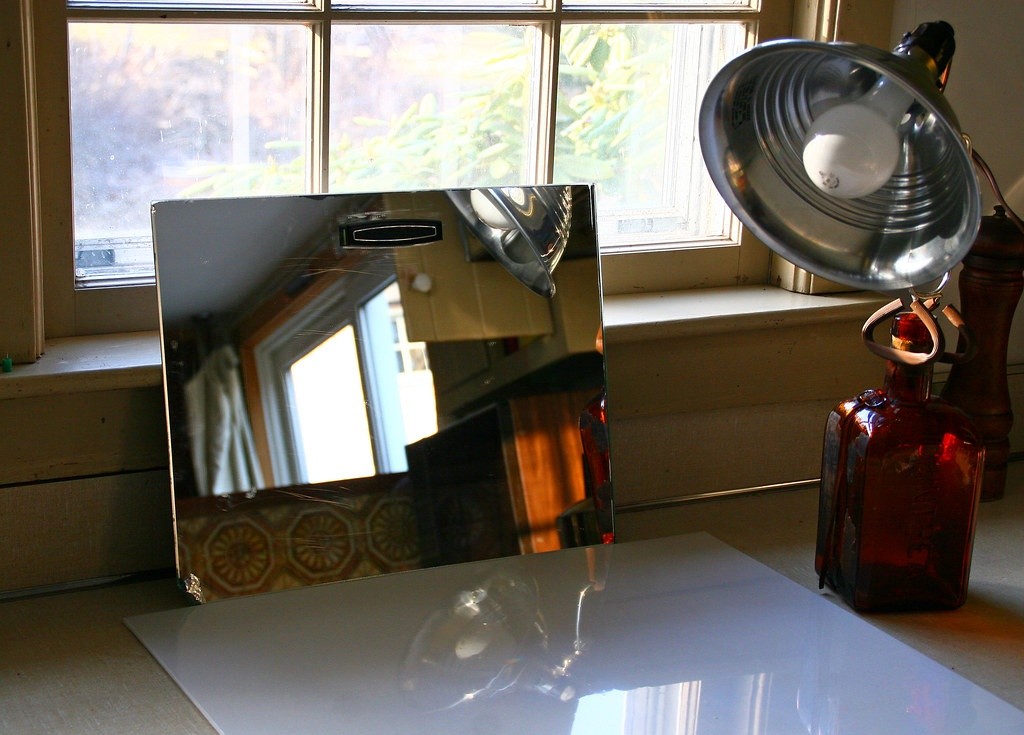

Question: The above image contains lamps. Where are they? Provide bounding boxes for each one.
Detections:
[697,20,1024,367]
[445,186,571,300]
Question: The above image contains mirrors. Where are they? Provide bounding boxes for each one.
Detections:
[150,185,616,602]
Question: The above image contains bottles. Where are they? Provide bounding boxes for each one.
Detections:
[814,312,985,612]
